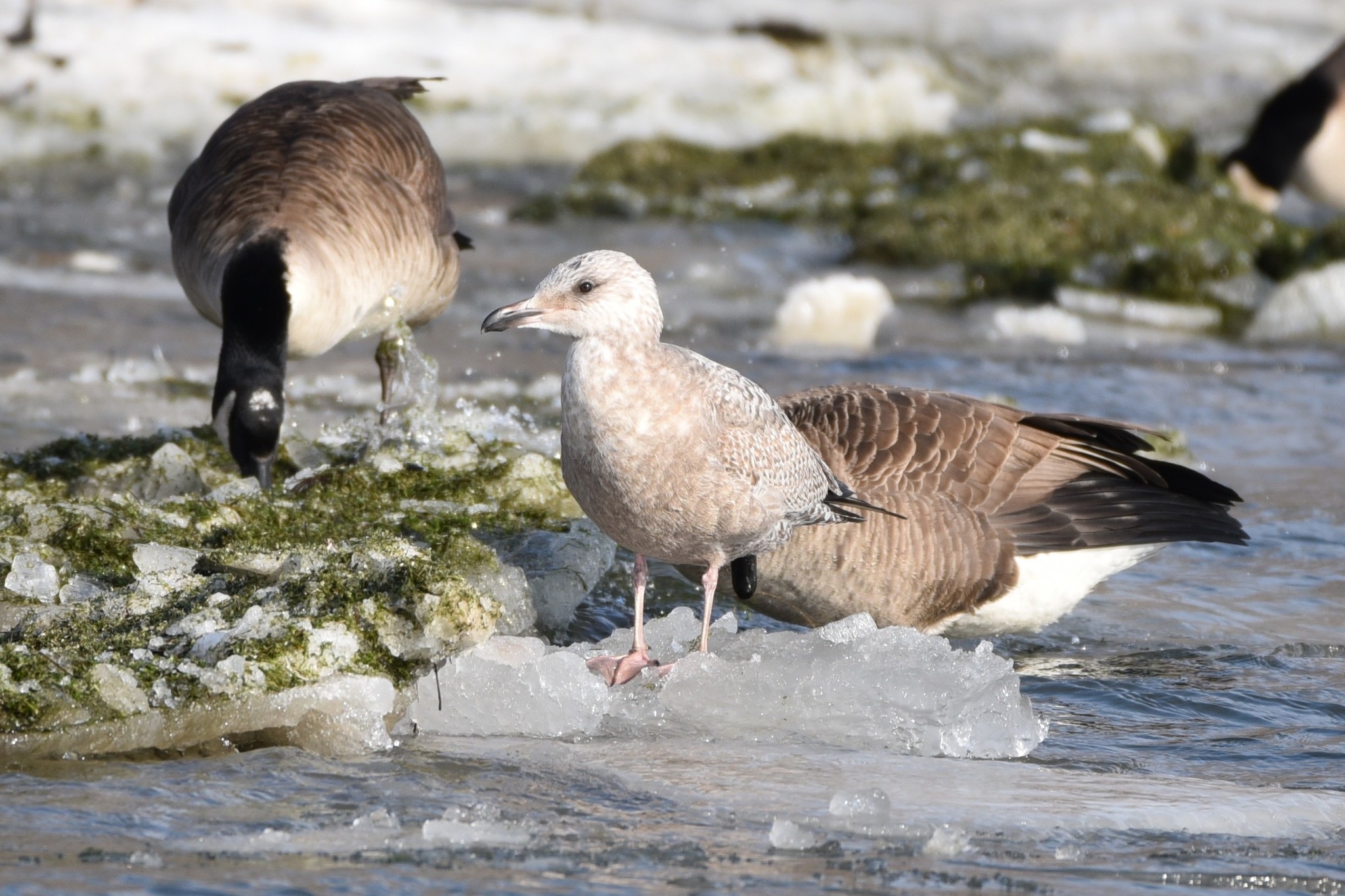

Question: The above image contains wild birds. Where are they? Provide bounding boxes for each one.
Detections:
[1214,34,1345,220]
[166,69,478,483]
[479,248,1251,686]
